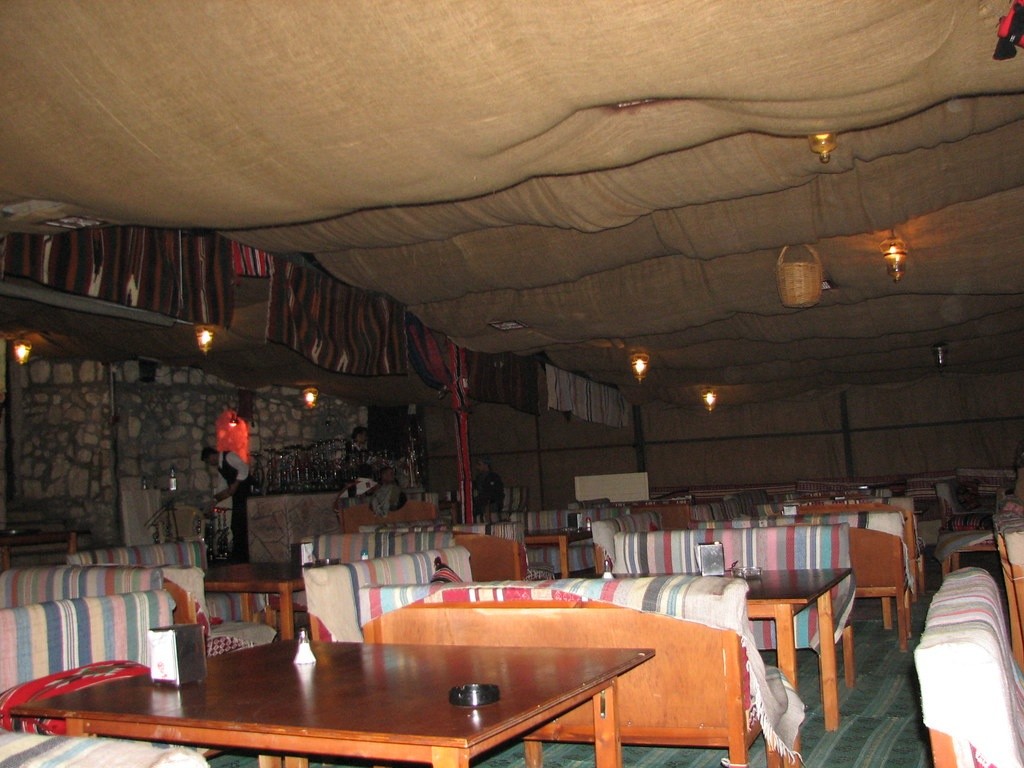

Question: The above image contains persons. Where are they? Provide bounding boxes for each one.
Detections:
[371,467,410,518]
[201,446,251,564]
[338,463,377,499]
[475,461,505,513]
[345,426,368,455]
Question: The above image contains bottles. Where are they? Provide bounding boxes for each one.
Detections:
[601,559,615,578]
[293,628,317,665]
[169,470,178,490]
[359,550,369,561]
[586,517,591,532]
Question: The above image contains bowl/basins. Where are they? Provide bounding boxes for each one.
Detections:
[731,566,763,580]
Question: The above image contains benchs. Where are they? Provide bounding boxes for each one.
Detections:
[0,472,1024,768]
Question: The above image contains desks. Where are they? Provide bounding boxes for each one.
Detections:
[940,532,999,577]
[198,562,305,643]
[8,642,655,768]
[595,567,858,767]
[527,528,592,579]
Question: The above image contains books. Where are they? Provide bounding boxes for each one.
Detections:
[781,506,798,515]
[694,541,724,577]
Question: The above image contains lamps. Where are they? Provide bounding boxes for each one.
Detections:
[13,331,32,365]
[195,326,215,356]
[302,384,320,409]
[879,227,909,285]
[809,131,838,165]
[697,386,719,413]
[629,345,654,379]
[932,341,949,374]
